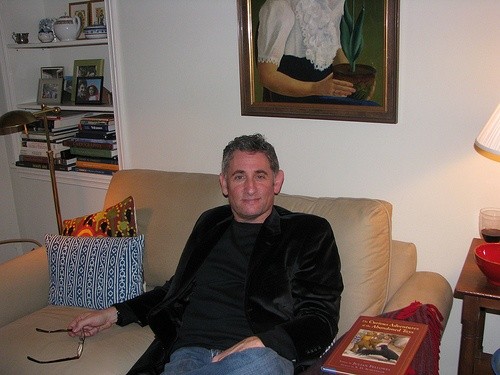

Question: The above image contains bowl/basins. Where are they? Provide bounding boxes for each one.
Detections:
[474,243,500,286]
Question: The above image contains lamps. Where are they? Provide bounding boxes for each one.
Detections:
[0,104,62,234]
[474,103,500,163]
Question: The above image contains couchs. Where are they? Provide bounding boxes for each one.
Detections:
[0,170,453,375]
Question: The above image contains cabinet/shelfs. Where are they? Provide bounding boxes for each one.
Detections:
[0,0,122,254]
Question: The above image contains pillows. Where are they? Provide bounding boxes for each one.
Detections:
[45,235,143,311]
[64,196,138,237]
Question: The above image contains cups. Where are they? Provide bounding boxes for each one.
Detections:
[479,207,500,243]
[12,32,29,44]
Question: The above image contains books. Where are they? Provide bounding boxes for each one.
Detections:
[14,110,119,177]
[320,314,430,374]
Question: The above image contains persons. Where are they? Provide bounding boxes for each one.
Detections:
[69,132,346,375]
[41,65,99,100]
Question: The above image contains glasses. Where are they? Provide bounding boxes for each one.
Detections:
[27,328,86,363]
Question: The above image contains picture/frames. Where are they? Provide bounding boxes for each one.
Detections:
[37,59,104,105]
[69,0,104,40]
[237,0,400,124]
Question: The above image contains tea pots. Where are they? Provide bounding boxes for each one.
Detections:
[38,24,56,43]
[53,12,81,41]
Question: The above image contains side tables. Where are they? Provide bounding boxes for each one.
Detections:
[453,238,500,375]
